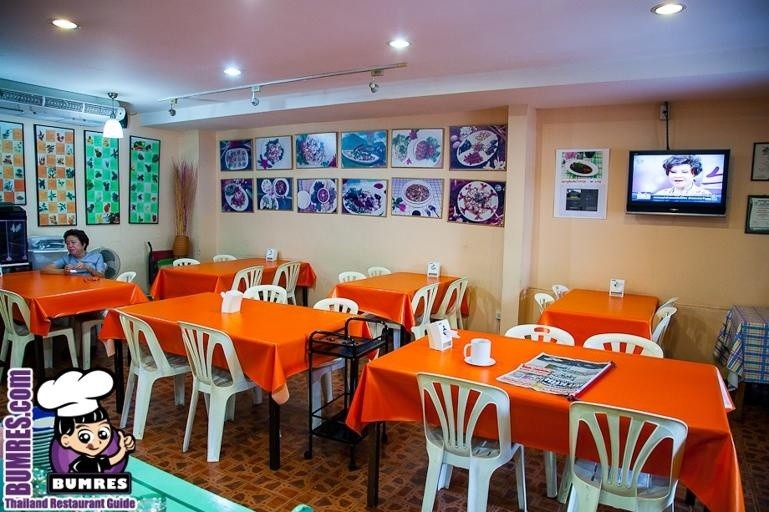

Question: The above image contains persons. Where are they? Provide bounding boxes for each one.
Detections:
[656,155,712,197]
[39,229,106,358]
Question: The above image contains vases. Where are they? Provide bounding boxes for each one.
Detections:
[172,236,190,259]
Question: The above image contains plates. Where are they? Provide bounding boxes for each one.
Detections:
[406,136,442,167]
[457,181,499,222]
[297,178,338,214]
[564,159,599,177]
[224,182,250,212]
[224,147,249,171]
[32,406,59,475]
[457,130,503,167]
[401,179,434,208]
[464,356,496,367]
[341,149,379,164]
[259,177,291,210]
[342,182,387,216]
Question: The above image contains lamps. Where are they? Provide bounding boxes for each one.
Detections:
[102,92,124,139]
[156,62,408,116]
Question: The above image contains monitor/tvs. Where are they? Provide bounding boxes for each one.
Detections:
[624,148,731,217]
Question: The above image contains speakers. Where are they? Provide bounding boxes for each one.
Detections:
[120,112,128,128]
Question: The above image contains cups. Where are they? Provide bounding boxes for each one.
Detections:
[464,338,492,364]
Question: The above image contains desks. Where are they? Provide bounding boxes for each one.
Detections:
[712,303,769,422]
[345,328,745,512]
